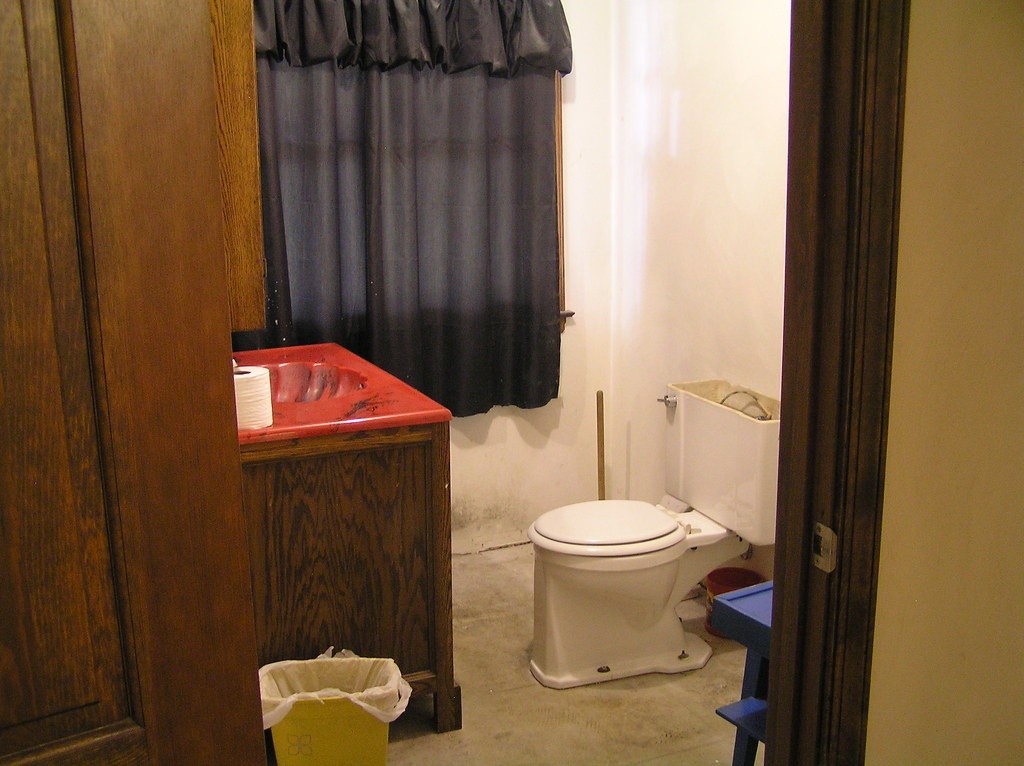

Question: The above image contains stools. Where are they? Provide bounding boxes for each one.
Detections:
[702,582,771,766]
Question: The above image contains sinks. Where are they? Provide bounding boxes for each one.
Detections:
[257,360,369,405]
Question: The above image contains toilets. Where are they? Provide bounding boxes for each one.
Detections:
[529,379,782,690]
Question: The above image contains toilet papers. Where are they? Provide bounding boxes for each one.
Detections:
[231,366,274,432]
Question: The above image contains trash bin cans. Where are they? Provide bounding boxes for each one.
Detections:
[259,648,411,766]
